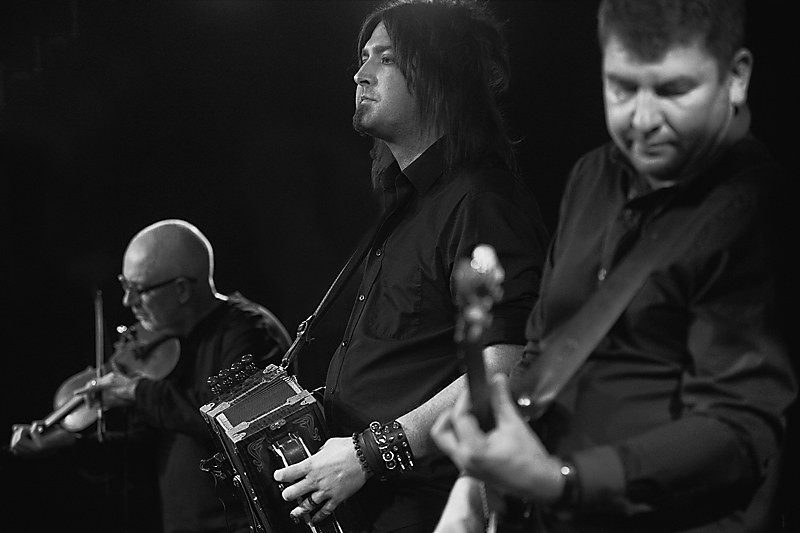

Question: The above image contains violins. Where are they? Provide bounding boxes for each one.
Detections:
[26,322,180,440]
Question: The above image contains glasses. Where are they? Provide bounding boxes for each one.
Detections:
[118,274,197,294]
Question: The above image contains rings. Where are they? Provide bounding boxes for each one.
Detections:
[308,496,319,507]
[320,509,331,516]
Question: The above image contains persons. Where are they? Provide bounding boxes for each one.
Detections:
[11,219,299,533]
[232,0,550,533]
[432,0,798,533]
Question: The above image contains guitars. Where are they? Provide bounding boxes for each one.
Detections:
[452,244,529,533]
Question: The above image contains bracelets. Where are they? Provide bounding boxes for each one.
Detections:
[362,421,416,486]
[548,466,573,509]
[351,432,370,473]
